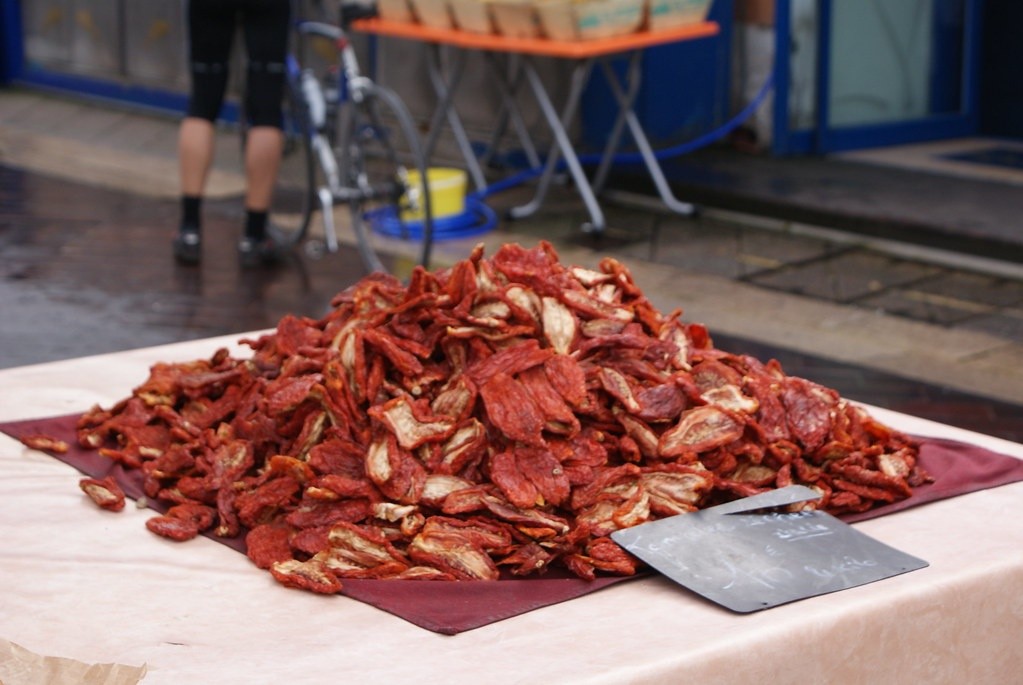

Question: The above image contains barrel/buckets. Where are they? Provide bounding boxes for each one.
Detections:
[400,167,468,220]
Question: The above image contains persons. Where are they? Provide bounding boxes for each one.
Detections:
[173,0,290,268]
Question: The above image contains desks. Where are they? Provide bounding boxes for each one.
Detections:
[353,16,719,238]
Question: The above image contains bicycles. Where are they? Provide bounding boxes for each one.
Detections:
[236,0,434,293]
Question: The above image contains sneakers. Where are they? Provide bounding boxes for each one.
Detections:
[237,234,291,267]
[172,228,203,264]
